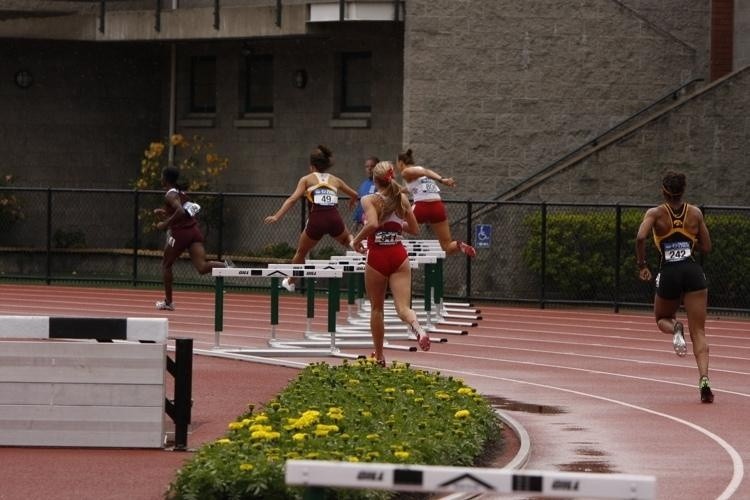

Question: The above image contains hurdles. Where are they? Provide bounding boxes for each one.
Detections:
[211,239,483,363]
[284,457,656,500]
[0,315,196,453]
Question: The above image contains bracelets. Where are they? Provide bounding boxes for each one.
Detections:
[637,259,647,265]
[439,176,444,183]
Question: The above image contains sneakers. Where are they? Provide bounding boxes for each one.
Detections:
[457,240,476,257]
[700,387,714,403]
[672,321,688,357]
[371,351,386,367]
[224,258,236,268]
[418,332,431,351]
[155,300,175,310]
[282,278,296,292]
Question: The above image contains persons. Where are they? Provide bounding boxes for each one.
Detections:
[263,144,360,293]
[153,166,239,311]
[635,171,715,405]
[396,148,476,258]
[347,155,389,300]
[353,159,431,369]
